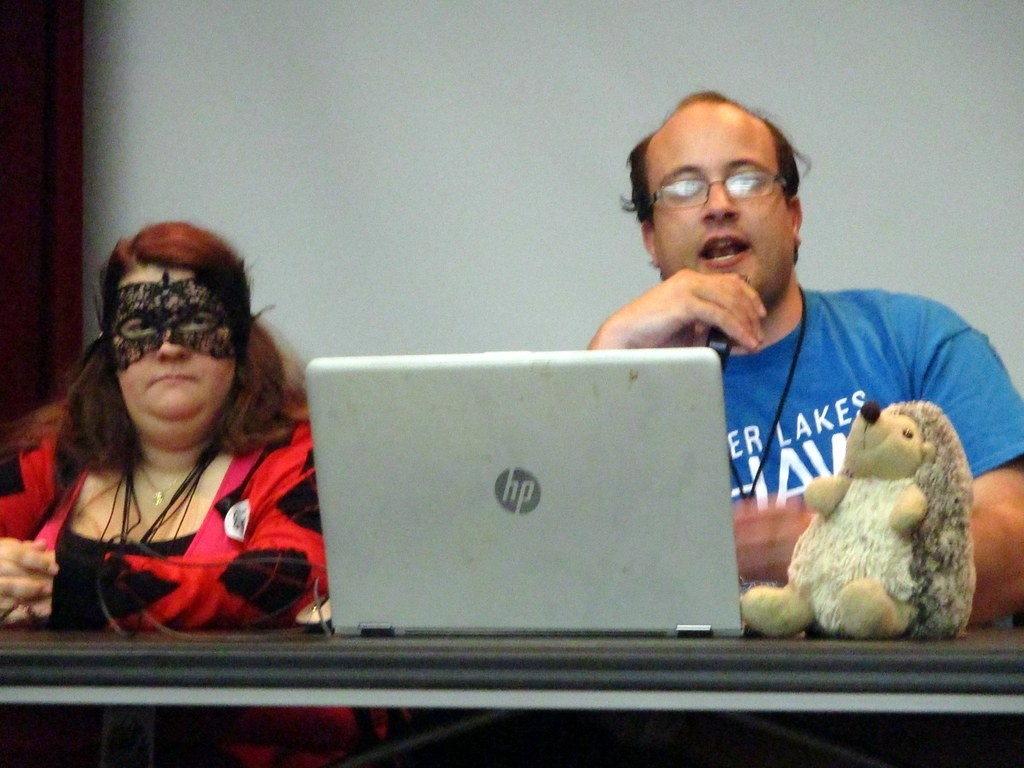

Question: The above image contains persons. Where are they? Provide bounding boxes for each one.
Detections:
[586,92,1024,625]
[0,221,409,768]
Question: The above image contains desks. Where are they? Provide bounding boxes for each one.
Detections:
[0,623,1024,715]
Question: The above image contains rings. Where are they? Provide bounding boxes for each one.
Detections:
[18,603,38,628]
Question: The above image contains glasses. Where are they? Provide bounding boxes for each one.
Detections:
[649,170,782,209]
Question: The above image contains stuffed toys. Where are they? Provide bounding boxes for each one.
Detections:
[738,400,977,642]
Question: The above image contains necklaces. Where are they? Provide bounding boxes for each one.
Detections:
[729,284,807,505]
[95,443,220,558]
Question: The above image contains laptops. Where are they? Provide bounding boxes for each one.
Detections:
[306,346,761,641]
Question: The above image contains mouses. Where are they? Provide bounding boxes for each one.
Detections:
[295,593,333,635]
